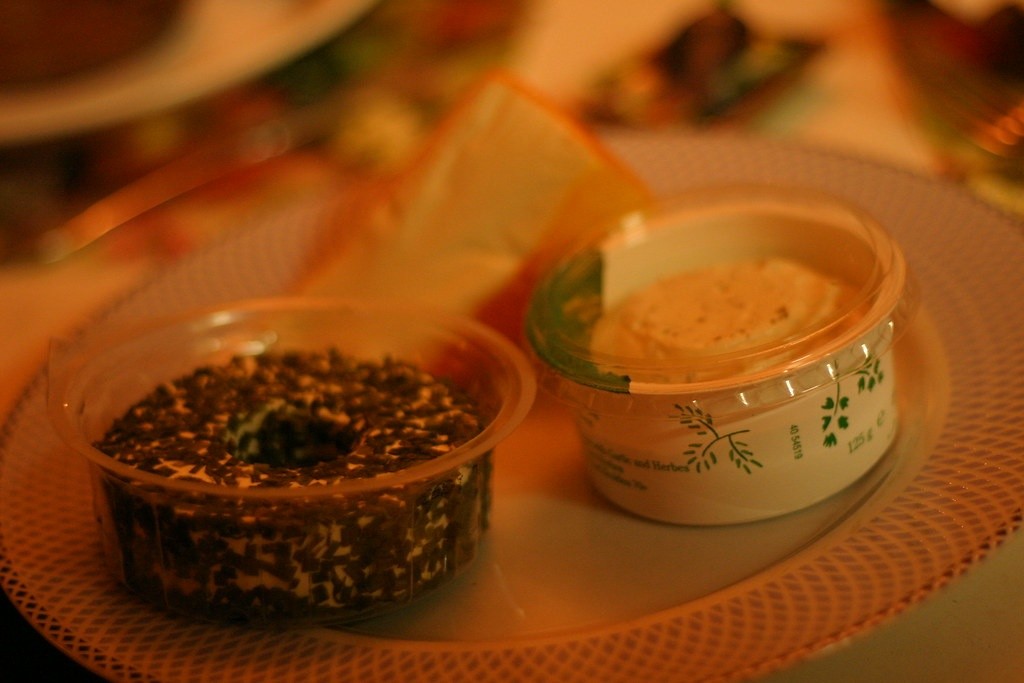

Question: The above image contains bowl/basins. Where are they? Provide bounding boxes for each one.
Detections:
[522,201,906,529]
[51,295,538,627]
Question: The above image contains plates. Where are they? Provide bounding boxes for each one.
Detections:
[0,0,384,146]
[0,133,1024,683]
